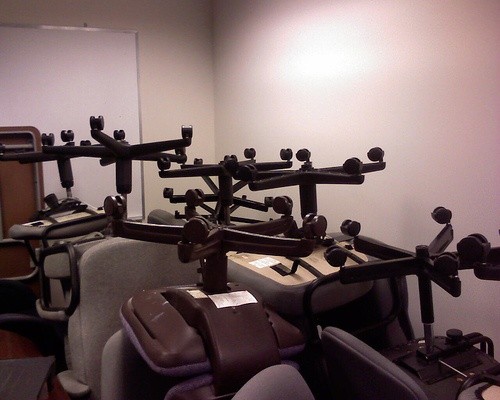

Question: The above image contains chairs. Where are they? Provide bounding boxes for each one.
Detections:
[0,115,500,400]
